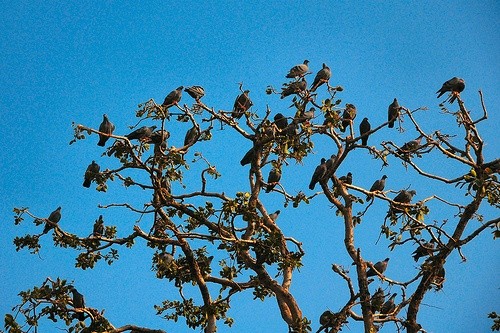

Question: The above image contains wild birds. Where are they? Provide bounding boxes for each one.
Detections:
[82,160,102,189]
[273,113,289,130]
[410,238,436,263]
[379,292,399,315]
[68,287,86,320]
[332,171,353,191]
[153,142,167,154]
[158,252,173,264]
[239,146,254,167]
[371,288,385,314]
[365,175,388,203]
[123,125,158,141]
[358,117,372,147]
[320,311,343,333]
[183,85,206,106]
[43,206,62,235]
[435,76,466,105]
[229,89,253,119]
[341,103,357,133]
[267,209,280,223]
[160,86,185,109]
[183,124,200,147]
[92,215,105,236]
[280,59,331,100]
[308,154,338,192]
[436,268,446,290]
[94,113,116,148]
[366,257,391,279]
[386,97,400,128]
[389,189,418,213]
[265,167,282,194]
[395,135,425,157]
[145,129,171,144]
[290,107,317,127]
